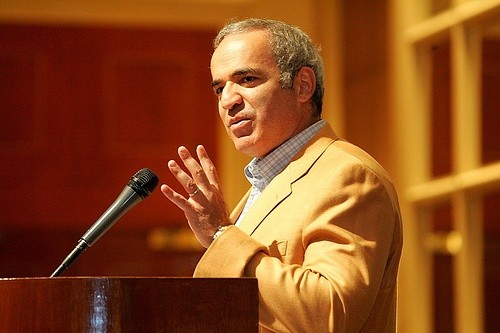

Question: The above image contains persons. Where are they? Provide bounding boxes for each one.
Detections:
[160,17,404,333]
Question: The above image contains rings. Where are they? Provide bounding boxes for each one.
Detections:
[188,186,200,197]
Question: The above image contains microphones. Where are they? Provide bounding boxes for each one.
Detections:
[51,167,159,277]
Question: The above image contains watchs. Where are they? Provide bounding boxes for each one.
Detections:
[212,224,234,241]
